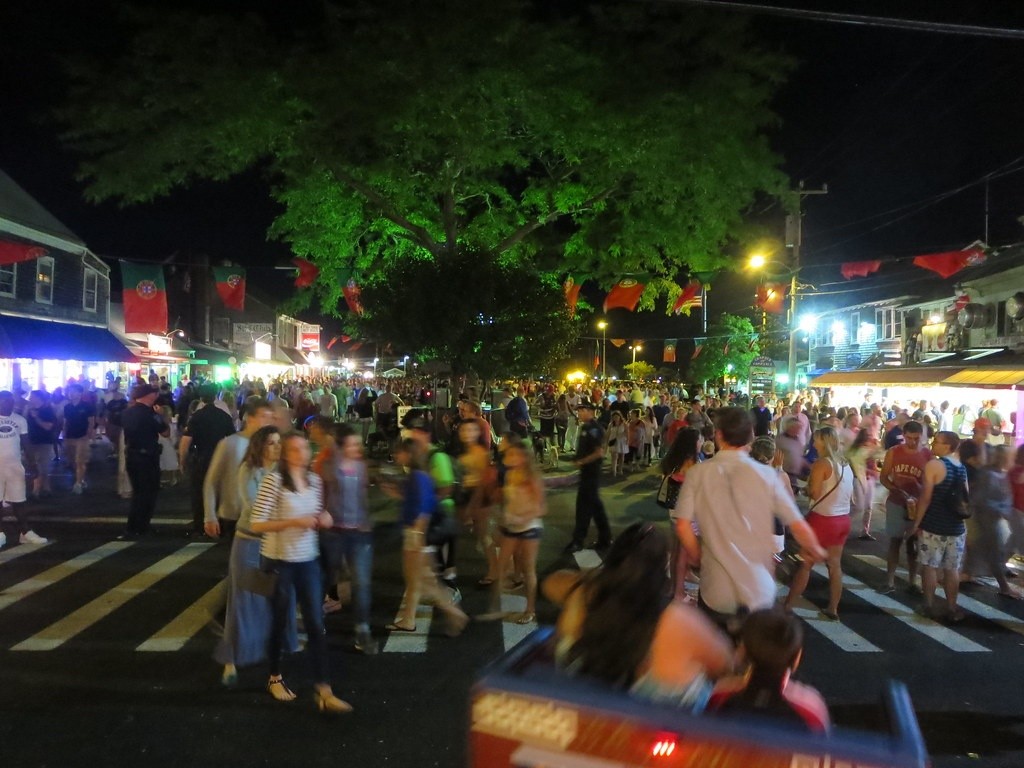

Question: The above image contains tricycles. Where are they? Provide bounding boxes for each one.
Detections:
[463,546,929,768]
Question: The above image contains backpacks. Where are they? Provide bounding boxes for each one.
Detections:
[934,470,973,519]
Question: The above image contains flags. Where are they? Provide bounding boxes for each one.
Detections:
[565,248,997,320]
[212,267,245,311]
[291,258,364,350]
[119,260,168,334]
[0,238,67,267]
[594,334,758,370]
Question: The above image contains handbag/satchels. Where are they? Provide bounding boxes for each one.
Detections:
[426,511,462,544]
[246,564,281,597]
[608,437,617,447]
[657,465,684,507]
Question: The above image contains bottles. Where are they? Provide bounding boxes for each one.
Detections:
[907,499,916,520]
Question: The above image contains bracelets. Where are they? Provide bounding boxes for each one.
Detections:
[412,530,424,534]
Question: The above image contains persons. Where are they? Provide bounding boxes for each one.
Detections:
[546,520,731,714]
[752,388,1024,622]
[703,607,829,739]
[673,407,829,629]
[659,428,700,606]
[496,378,749,477]
[202,392,549,713]
[0,369,234,546]
[214,374,434,447]
[562,402,611,554]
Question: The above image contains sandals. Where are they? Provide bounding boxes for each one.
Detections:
[315,692,354,714]
[266,679,297,704]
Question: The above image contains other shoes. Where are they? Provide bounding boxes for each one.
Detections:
[880,570,1024,601]
[821,607,839,623]
[916,604,966,621]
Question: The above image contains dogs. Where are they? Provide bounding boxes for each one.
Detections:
[532,431,545,464]
[547,446,559,468]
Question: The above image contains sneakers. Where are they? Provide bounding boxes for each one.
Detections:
[19,529,48,544]
[0,530,6,546]
[72,482,84,494]
[322,596,343,613]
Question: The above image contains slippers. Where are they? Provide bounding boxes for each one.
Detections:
[477,577,499,587]
[384,621,418,632]
[519,611,536,623]
[860,533,877,541]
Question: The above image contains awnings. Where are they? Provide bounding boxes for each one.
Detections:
[0,313,140,363]
[279,347,310,365]
[808,368,963,387]
[940,365,1024,392]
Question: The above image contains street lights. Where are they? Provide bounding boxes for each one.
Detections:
[628,345,641,381]
[404,356,410,378]
[373,358,379,378]
[749,255,797,395]
[598,320,607,388]
